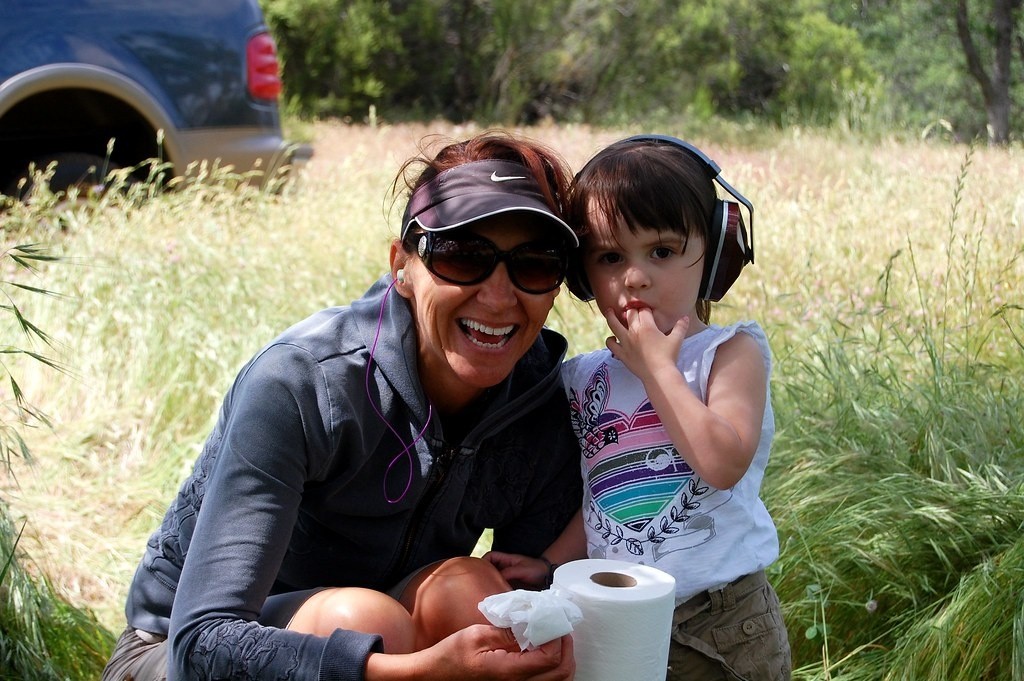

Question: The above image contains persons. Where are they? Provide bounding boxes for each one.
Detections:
[480,140,793,681]
[98,136,583,681]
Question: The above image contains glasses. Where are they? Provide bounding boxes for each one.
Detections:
[407,228,576,295]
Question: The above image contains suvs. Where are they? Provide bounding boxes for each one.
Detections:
[2,0,312,220]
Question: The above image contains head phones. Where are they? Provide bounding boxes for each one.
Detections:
[561,135,755,302]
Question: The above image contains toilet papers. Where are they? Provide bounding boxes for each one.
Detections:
[552,558,674,681]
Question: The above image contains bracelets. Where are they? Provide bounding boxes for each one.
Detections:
[536,554,558,576]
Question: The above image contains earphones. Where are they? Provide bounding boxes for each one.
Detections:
[397,269,404,283]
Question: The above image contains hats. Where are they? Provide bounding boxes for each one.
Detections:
[400,161,581,251]
